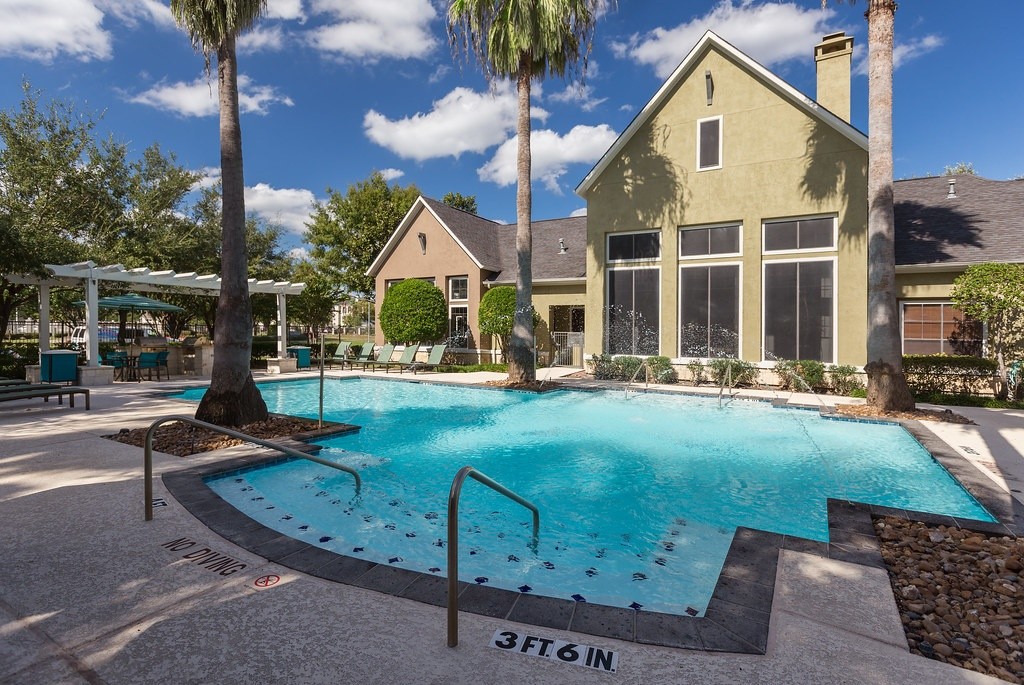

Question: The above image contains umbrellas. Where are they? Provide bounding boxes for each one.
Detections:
[71,293,186,379]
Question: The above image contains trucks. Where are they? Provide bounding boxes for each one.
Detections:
[70,326,159,344]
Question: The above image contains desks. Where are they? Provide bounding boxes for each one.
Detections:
[111,356,139,381]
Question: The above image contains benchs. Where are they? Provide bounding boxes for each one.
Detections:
[0,377,90,410]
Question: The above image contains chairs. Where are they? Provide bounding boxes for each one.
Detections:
[97,352,170,383]
[317,342,447,374]
